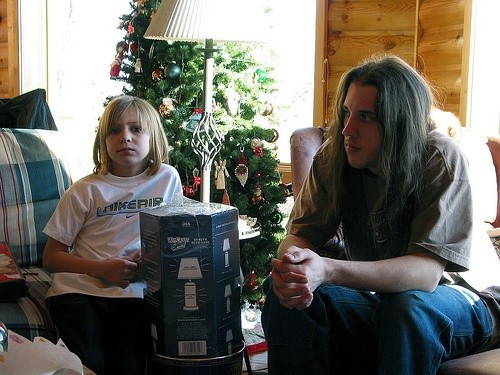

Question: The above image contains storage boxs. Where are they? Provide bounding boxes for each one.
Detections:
[140,203,246,375]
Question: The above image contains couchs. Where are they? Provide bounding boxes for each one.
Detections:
[0,129,74,344]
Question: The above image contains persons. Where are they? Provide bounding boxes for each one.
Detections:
[42,96,185,375]
[260,56,500,375]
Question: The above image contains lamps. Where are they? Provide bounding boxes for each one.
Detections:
[144,0,270,202]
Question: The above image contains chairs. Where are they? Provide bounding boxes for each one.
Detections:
[290,125,500,375]
